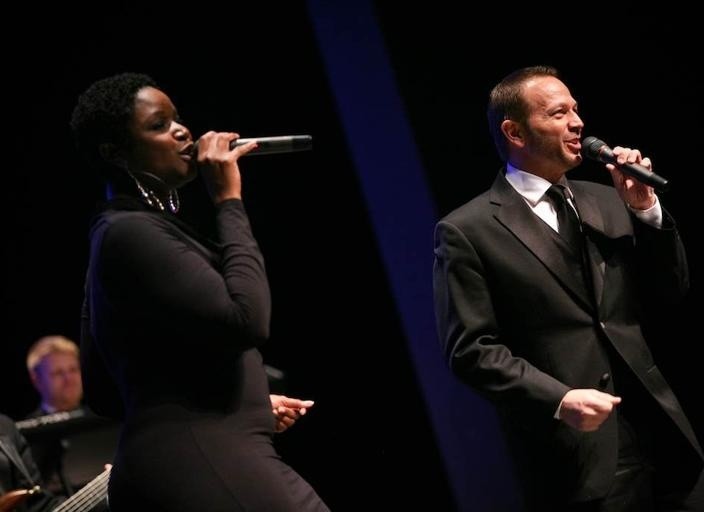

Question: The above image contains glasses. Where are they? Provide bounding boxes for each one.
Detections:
[35,367,80,375]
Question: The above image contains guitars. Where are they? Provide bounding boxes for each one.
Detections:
[0,468,110,511]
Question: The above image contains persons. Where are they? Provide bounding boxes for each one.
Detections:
[71,73,332,512]
[431,66,704,512]
[0,335,90,512]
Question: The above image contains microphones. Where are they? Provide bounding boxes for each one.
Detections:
[193,134,314,158]
[581,136,668,191]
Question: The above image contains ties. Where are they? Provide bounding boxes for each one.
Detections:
[545,185,583,251]
